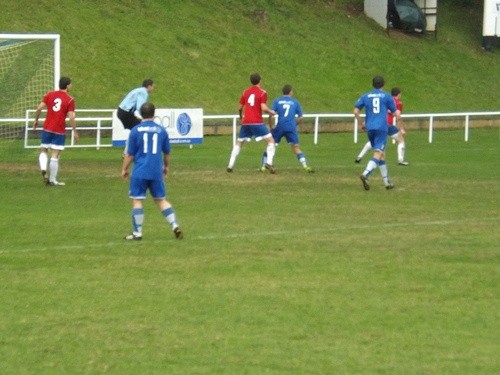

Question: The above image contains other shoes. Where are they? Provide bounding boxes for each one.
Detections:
[173,226,182,240]
[360,174,370,190]
[48,181,64,185]
[265,164,275,174]
[399,161,408,166]
[41,169,48,183]
[260,167,267,172]
[227,167,232,174]
[304,167,313,173]
[385,181,394,189]
[355,157,361,163]
[123,231,142,241]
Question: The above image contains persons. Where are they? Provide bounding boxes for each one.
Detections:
[260,85,315,173]
[116,79,154,158]
[226,72,278,174]
[121,102,184,239]
[33,77,81,185]
[353,75,395,190]
[355,87,410,166]
